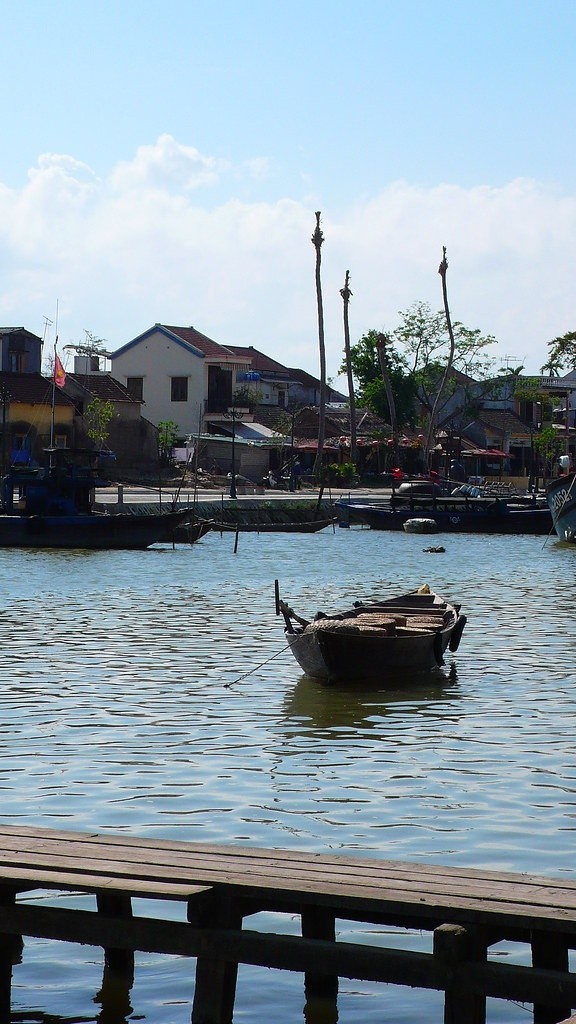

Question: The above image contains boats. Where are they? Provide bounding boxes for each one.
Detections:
[213,516,337,533]
[332,496,556,533]
[545,472,576,545]
[159,519,216,543]
[275,579,468,686]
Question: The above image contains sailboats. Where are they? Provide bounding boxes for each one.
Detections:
[0,336,193,551]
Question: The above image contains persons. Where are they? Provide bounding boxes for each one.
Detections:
[293,460,302,490]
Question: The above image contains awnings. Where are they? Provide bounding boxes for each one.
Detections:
[211,422,507,457]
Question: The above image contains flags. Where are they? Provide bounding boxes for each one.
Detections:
[55,356,66,388]
